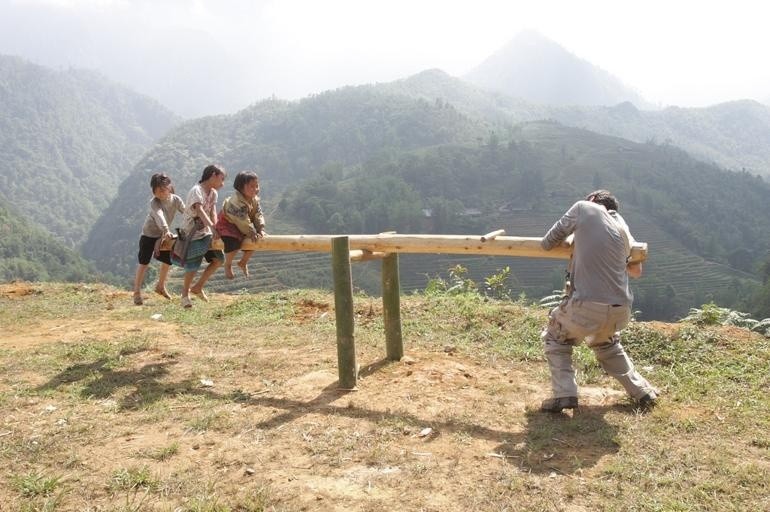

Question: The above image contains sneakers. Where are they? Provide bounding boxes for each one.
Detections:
[541,396,578,411]
[640,388,661,406]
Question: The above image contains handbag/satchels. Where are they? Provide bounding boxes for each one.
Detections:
[154,236,189,268]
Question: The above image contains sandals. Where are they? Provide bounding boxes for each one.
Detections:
[154,286,171,300]
[134,293,143,305]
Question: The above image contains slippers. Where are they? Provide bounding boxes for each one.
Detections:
[181,292,192,309]
[191,288,208,302]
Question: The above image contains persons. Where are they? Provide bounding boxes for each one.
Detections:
[214,170,269,281]
[540,189,659,415]
[171,164,227,310]
[131,170,186,306]
